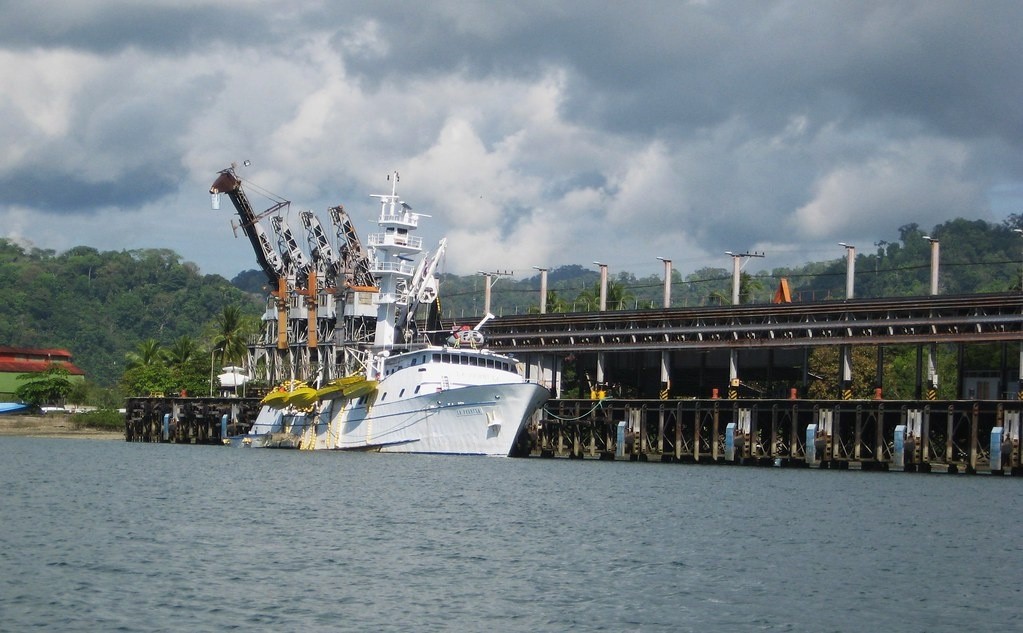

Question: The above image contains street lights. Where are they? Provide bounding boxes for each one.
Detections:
[210,347,224,398]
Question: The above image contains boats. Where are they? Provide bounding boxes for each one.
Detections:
[259,374,379,416]
[207,161,552,460]
[446,321,484,348]
